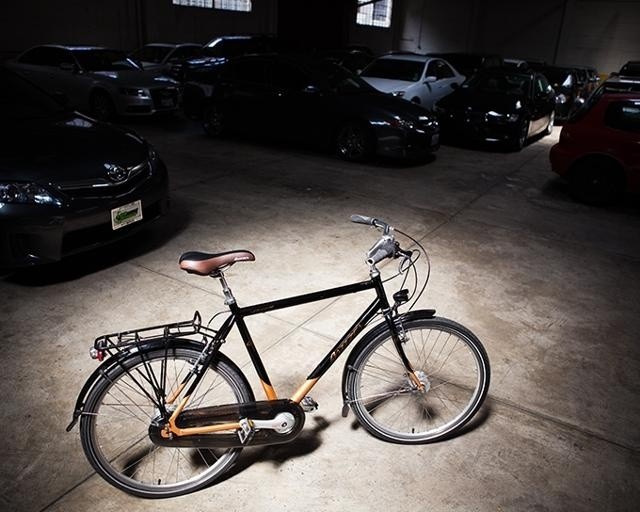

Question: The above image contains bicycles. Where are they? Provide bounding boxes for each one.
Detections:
[66,214,491,499]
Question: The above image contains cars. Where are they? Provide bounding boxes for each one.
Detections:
[5,43,185,126]
[431,58,639,214]
[0,69,169,281]
